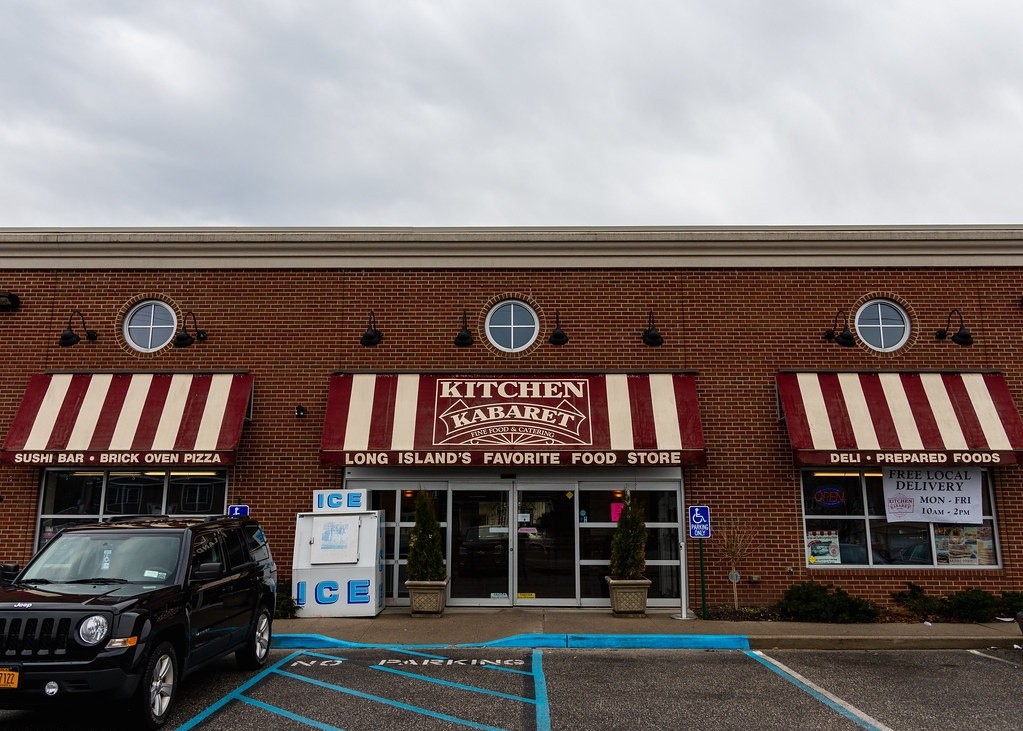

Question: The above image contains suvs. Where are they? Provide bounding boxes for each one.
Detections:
[0,513,278,729]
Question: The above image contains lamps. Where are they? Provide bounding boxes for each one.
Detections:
[933,308,978,347]
[60,306,97,351]
[642,308,664,346]
[453,308,476,347]
[358,310,384,349]
[550,308,569,345]
[174,309,207,348]
[822,308,857,345]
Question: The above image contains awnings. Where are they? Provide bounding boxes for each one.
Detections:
[2,371,256,469]
[317,369,708,469]
[775,369,1023,466]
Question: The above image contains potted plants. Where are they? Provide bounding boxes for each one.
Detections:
[402,484,450,619]
[601,487,652,619]
